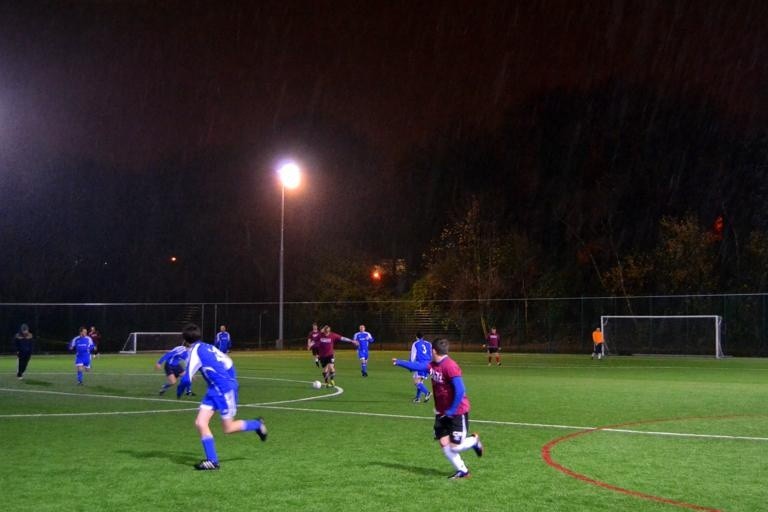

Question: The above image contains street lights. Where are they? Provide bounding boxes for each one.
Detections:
[274,161,301,350]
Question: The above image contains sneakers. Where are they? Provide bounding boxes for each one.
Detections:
[448,471,471,479]
[256,417,267,440]
[413,399,421,403]
[424,392,432,402]
[194,460,219,470]
[472,433,483,456]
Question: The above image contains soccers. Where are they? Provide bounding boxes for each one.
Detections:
[312,381,321,388]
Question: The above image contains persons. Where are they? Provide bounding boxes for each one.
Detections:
[155,340,197,396]
[391,337,484,480]
[408,330,434,404]
[12,324,35,381]
[307,322,374,387]
[176,321,269,471]
[66,326,97,386]
[482,325,504,368]
[213,324,233,354]
[87,326,102,360]
[590,325,606,362]
